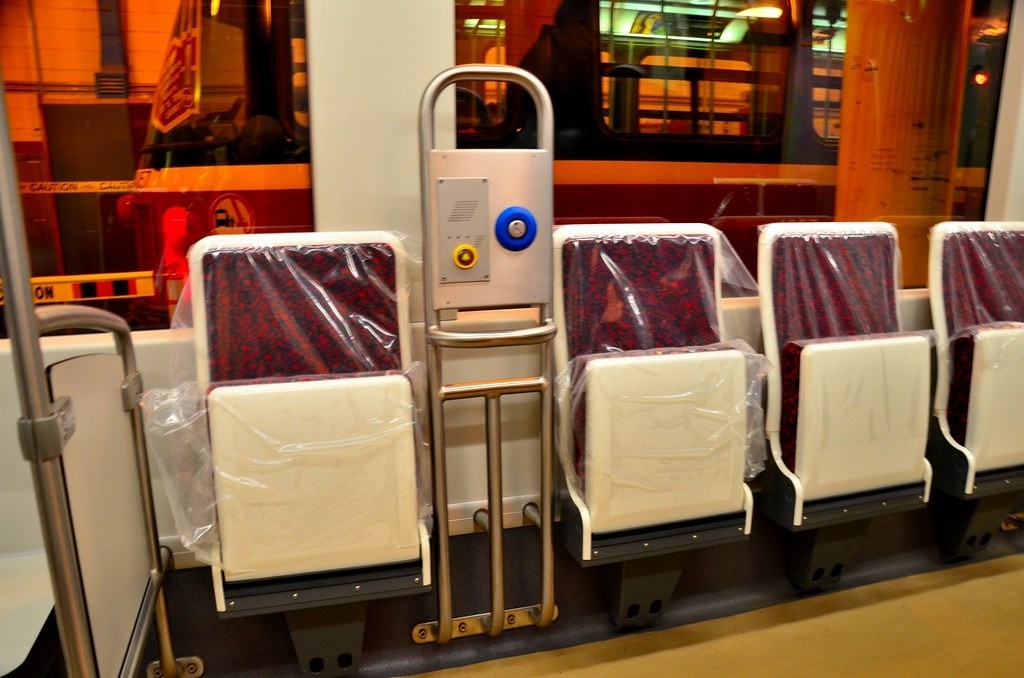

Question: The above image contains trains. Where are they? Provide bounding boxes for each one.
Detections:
[117,0,1012,332]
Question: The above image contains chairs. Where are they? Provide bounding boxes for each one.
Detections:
[559,224,755,626]
[926,219,1024,563]
[187,235,438,678]
[748,218,936,594]
